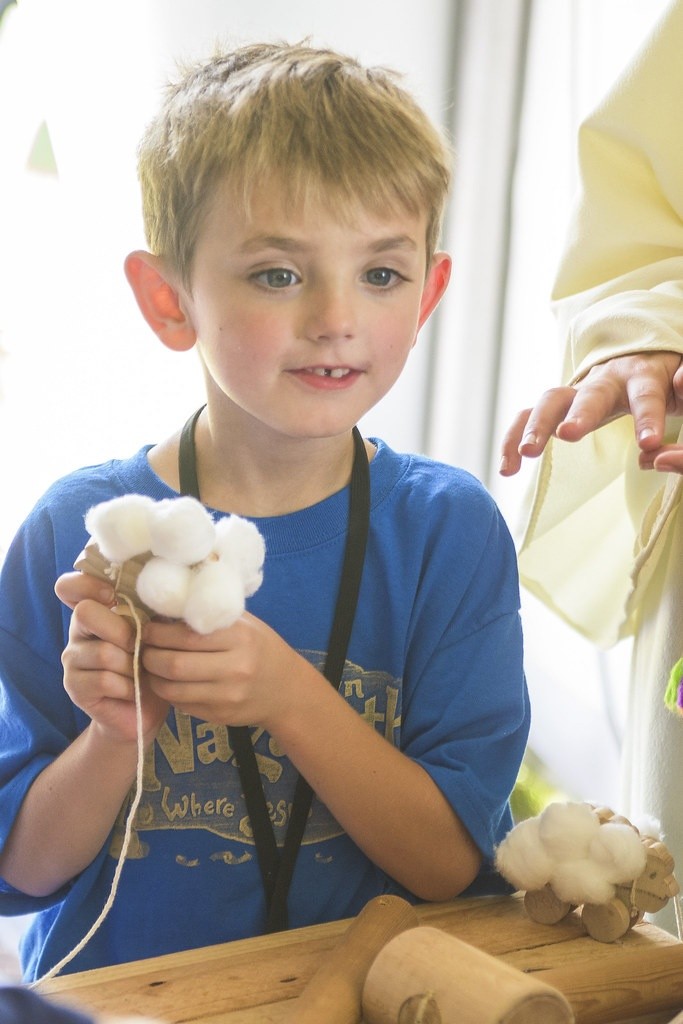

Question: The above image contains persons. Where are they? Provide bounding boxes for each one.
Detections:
[499,0,683,956]
[0,35,530,1024]
